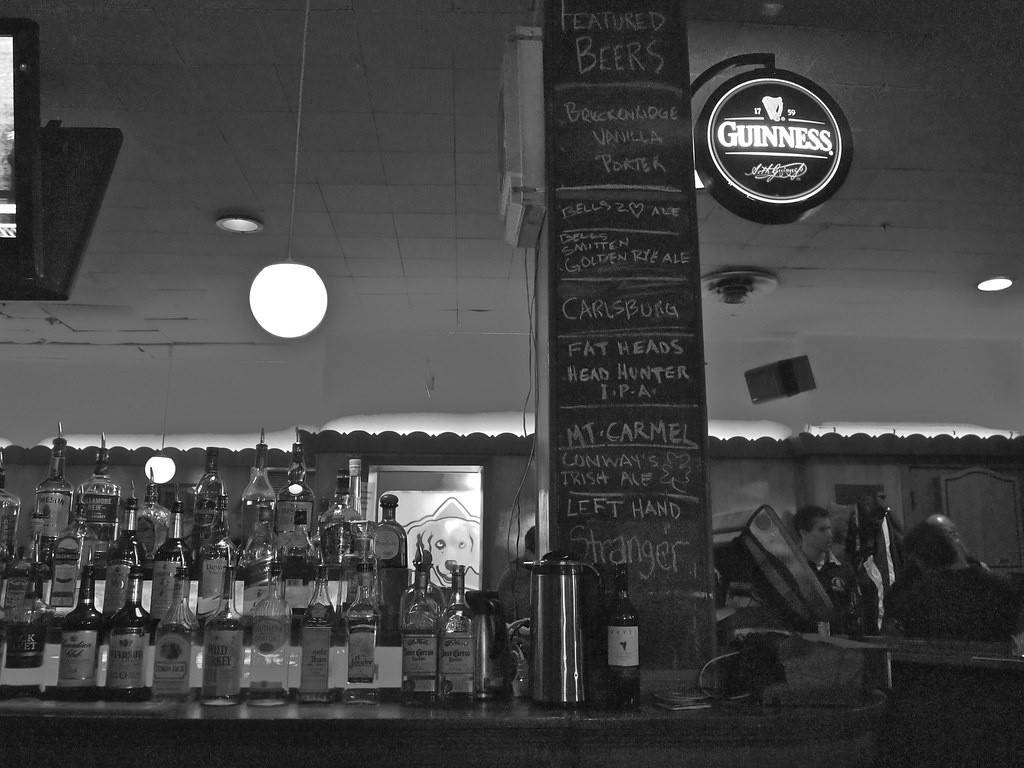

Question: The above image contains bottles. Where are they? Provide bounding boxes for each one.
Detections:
[56,563,104,700]
[105,566,150,702]
[606,565,643,710]
[440,565,476,706]
[400,563,439,707]
[296,564,334,703]
[0,563,47,697]
[342,563,381,704]
[249,568,290,708]
[152,570,195,703]
[0,438,409,646]
[201,563,244,707]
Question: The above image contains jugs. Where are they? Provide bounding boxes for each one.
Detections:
[522,549,604,709]
[467,588,516,702]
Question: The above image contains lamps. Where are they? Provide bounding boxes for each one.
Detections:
[144,343,176,484]
[249,0,327,338]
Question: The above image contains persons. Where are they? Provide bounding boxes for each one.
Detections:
[882,516,1017,768]
[499,527,536,623]
[793,505,855,612]
